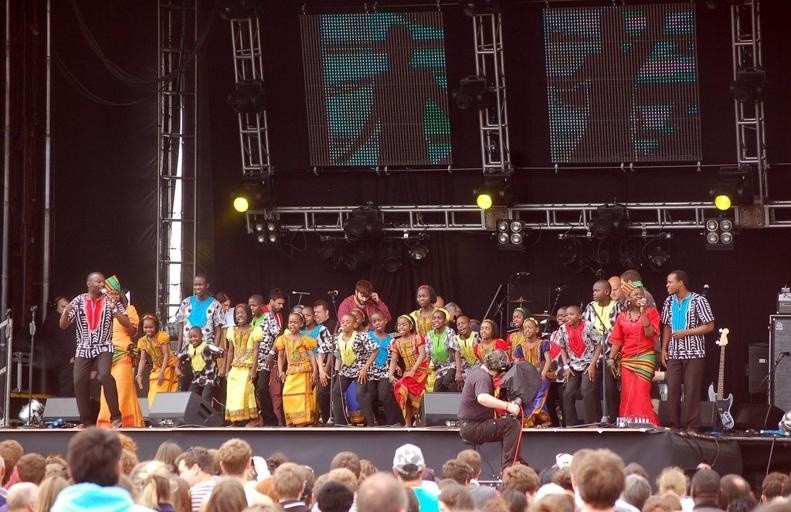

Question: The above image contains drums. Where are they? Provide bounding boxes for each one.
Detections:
[538,317,559,336]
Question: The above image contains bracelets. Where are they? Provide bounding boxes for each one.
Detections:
[643,322,653,327]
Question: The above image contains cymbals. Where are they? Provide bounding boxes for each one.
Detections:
[507,297,533,307]
[533,312,556,321]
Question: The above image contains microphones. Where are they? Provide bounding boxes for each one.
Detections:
[31,305,38,311]
[329,290,338,296]
[555,285,568,292]
[512,397,521,420]
[6,309,12,316]
[293,291,311,295]
[101,289,117,304]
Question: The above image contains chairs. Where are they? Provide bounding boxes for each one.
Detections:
[179,354,227,411]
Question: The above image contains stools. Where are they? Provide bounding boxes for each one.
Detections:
[459,425,503,487]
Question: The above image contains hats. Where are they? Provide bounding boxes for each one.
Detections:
[393,444,426,477]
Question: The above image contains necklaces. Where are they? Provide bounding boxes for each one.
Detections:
[629,308,641,320]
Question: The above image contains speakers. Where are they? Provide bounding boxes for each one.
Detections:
[768,315,790,413]
[423,389,472,429]
[744,342,768,392]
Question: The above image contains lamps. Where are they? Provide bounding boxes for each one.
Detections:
[707,175,754,210]
[705,217,736,252]
[496,218,526,252]
[253,220,285,250]
[474,170,515,211]
[230,176,271,213]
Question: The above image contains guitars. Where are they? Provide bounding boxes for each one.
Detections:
[707,327,736,433]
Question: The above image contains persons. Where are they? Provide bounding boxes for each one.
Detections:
[98,274,144,429]
[454,449,483,489]
[443,460,474,487]
[642,490,683,512]
[114,434,140,475]
[389,445,441,512]
[138,469,174,512]
[215,440,270,506]
[60,272,131,429]
[134,313,179,410]
[347,307,369,336]
[127,462,167,496]
[550,305,568,419]
[574,445,631,512]
[583,278,627,424]
[317,482,355,512]
[689,468,724,512]
[312,301,338,334]
[201,477,247,512]
[618,460,648,485]
[37,477,67,512]
[262,294,287,335]
[435,482,476,512]
[151,438,182,469]
[515,315,556,429]
[14,454,47,486]
[314,314,380,427]
[176,276,226,349]
[40,454,70,486]
[458,350,524,480]
[657,466,696,512]
[536,493,577,512]
[537,465,574,494]
[176,446,218,512]
[389,314,431,428]
[473,319,511,396]
[225,303,264,428]
[409,286,450,338]
[761,472,790,505]
[44,428,158,512]
[295,305,331,363]
[275,313,320,428]
[40,297,75,398]
[215,292,235,326]
[270,463,310,511]
[244,296,281,425]
[3,483,41,512]
[363,313,400,427]
[618,270,660,371]
[606,278,630,313]
[444,303,461,321]
[622,475,651,512]
[176,326,226,412]
[717,473,749,508]
[323,468,356,492]
[0,439,22,489]
[453,315,480,367]
[336,282,392,333]
[508,306,531,352]
[556,306,602,429]
[498,465,538,505]
[425,309,470,392]
[618,270,655,316]
[327,451,360,477]
[662,267,714,434]
[261,448,288,476]
[355,473,409,512]
